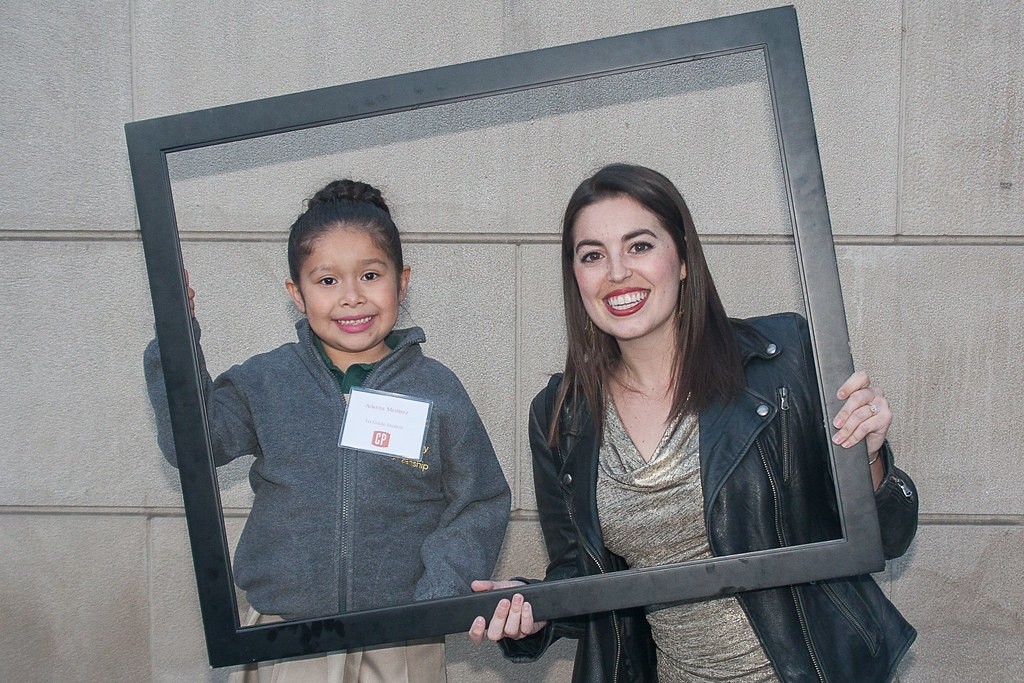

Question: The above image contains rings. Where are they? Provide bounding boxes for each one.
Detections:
[865,402,878,417]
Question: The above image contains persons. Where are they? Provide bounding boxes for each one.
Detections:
[140,178,515,683]
[467,163,922,683]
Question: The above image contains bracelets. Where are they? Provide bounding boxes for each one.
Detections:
[868,449,880,465]
